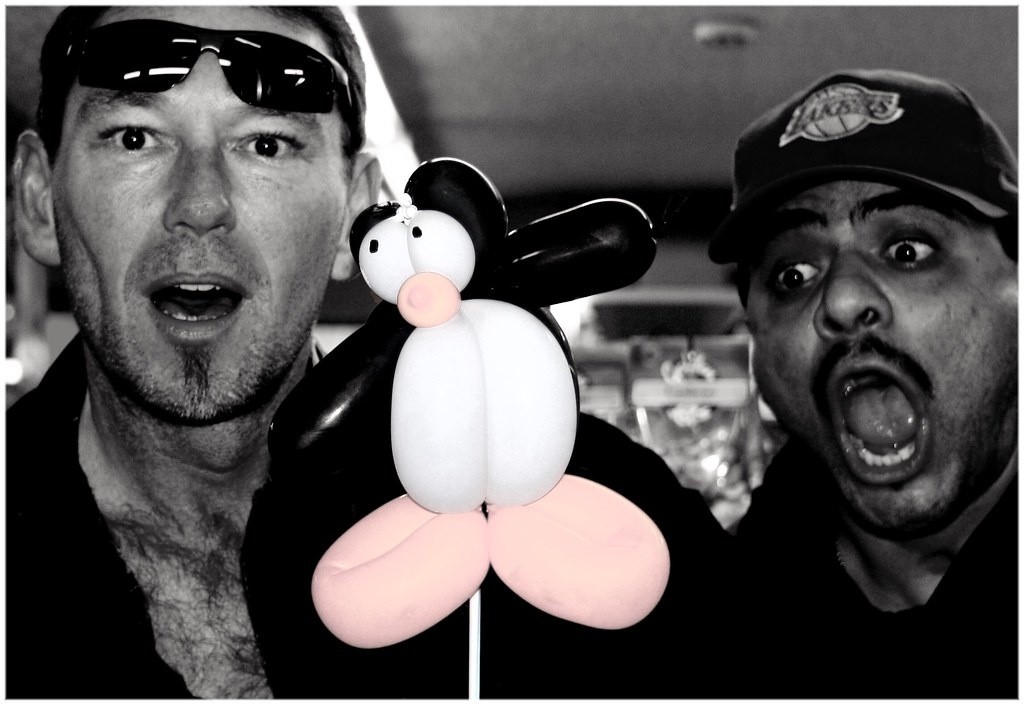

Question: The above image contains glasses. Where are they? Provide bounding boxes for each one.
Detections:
[44,19,357,143]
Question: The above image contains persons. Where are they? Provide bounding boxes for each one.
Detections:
[705,70,1017,700]
[6,6,745,699]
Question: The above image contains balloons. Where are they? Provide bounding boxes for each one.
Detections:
[264,157,670,648]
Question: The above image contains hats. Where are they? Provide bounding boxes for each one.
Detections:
[708,69,1018,265]
